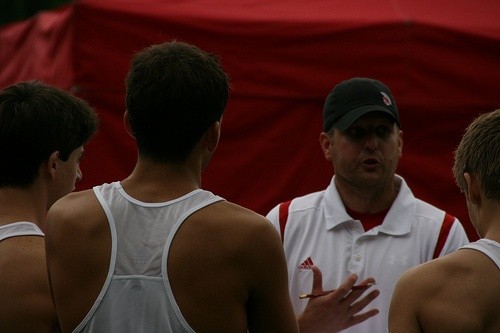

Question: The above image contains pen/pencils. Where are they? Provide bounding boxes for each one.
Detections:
[299,283,373,301]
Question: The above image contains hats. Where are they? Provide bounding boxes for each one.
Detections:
[323,75,403,139]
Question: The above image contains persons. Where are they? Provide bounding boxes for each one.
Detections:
[43,41,301,333]
[1,78,99,333]
[386,107,500,333]
[262,77,471,333]
